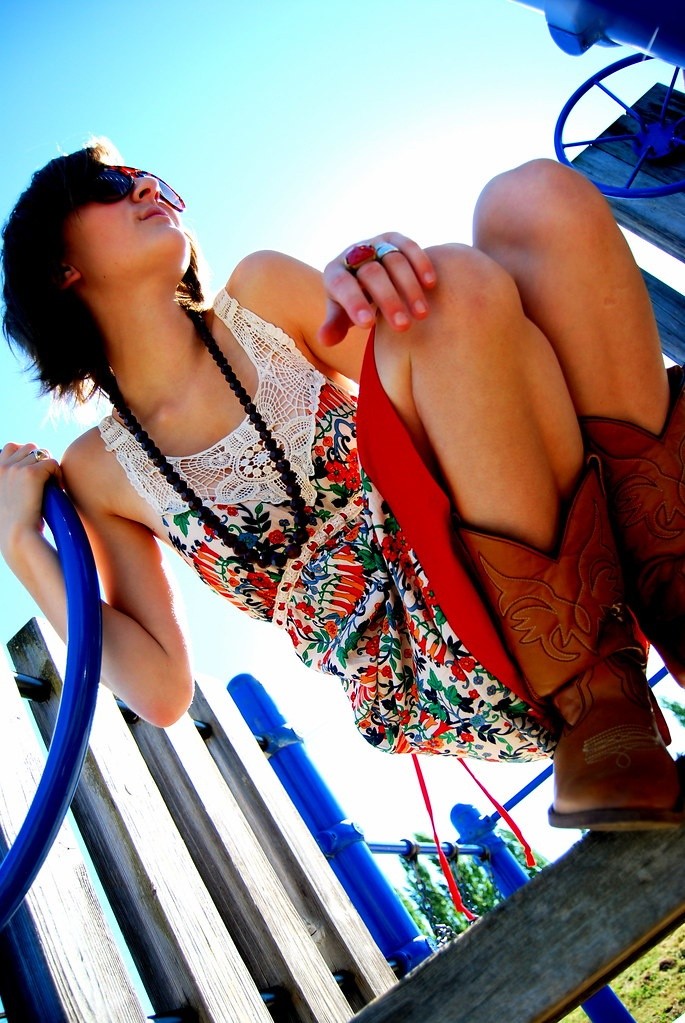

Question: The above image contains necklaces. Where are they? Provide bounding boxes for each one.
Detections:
[99,305,309,568]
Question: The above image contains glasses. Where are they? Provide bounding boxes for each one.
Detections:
[70,167,185,215]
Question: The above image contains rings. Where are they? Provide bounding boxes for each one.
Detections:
[28,449,48,462]
[372,241,400,260]
[344,243,376,272]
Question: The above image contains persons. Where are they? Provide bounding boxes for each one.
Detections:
[0,142,684,829]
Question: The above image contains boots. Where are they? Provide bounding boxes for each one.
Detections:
[451,455,685,831]
[580,362,685,688]
